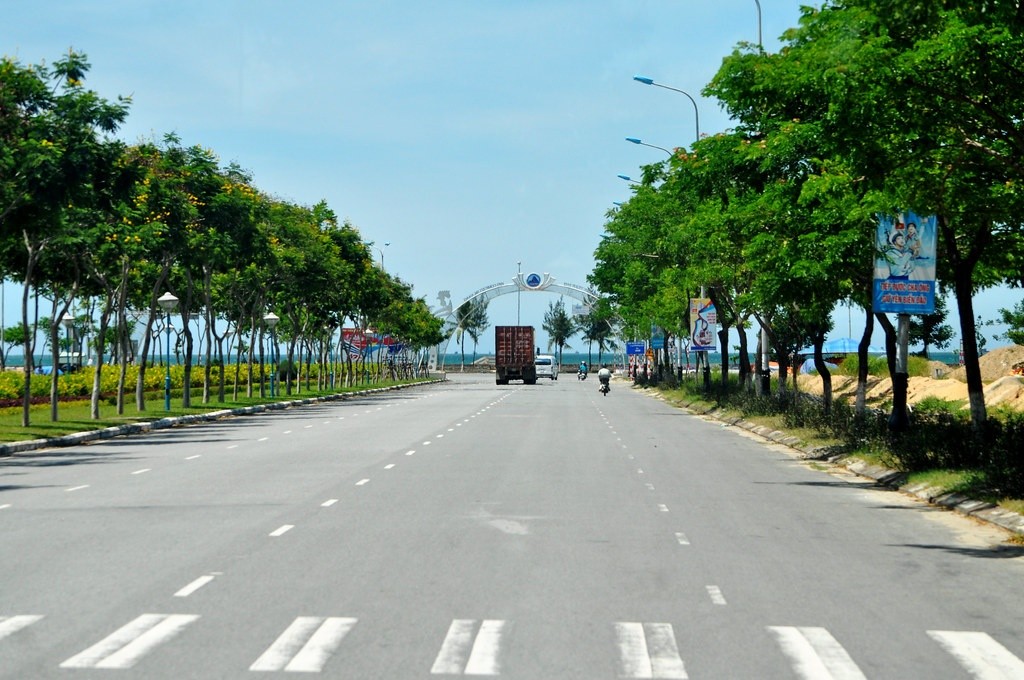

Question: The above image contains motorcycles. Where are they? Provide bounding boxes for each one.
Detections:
[577,369,588,380]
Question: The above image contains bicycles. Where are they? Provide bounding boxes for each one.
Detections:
[599,379,610,396]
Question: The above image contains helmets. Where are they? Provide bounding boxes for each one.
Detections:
[581,361,586,365]
[602,363,606,367]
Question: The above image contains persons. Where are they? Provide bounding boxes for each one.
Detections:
[578,360,587,379]
[885,217,928,281]
[597,362,611,391]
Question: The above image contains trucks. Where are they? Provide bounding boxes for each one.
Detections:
[492,324,541,385]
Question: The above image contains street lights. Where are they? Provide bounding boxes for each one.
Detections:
[361,328,374,385]
[324,324,336,390]
[60,314,76,374]
[262,311,282,397]
[623,136,686,388]
[617,173,675,374]
[222,331,234,354]
[632,76,711,396]
[364,240,390,377]
[156,291,180,412]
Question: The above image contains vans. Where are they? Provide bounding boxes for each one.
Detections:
[535,354,561,381]
